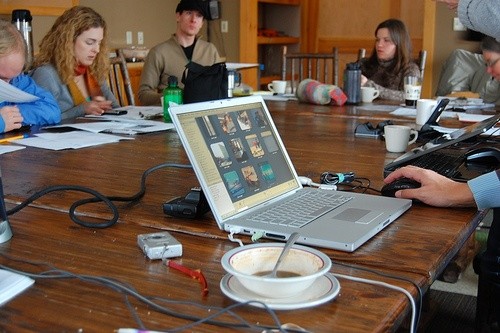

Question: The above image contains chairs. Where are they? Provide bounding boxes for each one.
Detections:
[281,45,339,94]
[105,49,135,108]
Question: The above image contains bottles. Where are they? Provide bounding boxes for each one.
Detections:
[342,62,361,102]
[163,75,183,123]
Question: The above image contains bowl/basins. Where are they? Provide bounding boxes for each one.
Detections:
[233,87,253,97]
[221,243,332,299]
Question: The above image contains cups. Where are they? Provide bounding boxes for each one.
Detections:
[404,75,423,100]
[384,125,418,152]
[360,87,379,104]
[268,79,288,94]
[415,99,440,125]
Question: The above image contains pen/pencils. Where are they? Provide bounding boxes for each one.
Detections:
[0,133,29,143]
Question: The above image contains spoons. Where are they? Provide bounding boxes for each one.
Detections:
[262,232,299,278]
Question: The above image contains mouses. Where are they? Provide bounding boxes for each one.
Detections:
[465,147,500,167]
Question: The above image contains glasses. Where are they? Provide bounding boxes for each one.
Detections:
[485,57,500,68]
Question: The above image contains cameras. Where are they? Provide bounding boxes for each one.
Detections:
[136,231,183,261]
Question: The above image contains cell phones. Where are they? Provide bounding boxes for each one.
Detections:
[103,110,128,116]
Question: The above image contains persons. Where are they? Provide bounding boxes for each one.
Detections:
[138,0,221,105]
[0,19,61,133]
[346,18,420,100]
[384,164,500,210]
[441,0,500,80]
[24,6,120,119]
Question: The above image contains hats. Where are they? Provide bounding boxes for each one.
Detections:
[176,0,211,21]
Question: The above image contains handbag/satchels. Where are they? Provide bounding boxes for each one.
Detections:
[180,61,229,105]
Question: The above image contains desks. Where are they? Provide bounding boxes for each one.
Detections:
[0,98,500,333]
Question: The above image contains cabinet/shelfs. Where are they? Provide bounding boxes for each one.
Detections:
[106,62,144,105]
[239,0,309,90]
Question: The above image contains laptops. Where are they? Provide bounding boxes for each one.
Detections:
[380,178,422,204]
[383,114,500,184]
[168,95,413,253]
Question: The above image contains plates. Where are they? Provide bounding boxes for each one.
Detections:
[220,273,341,310]
